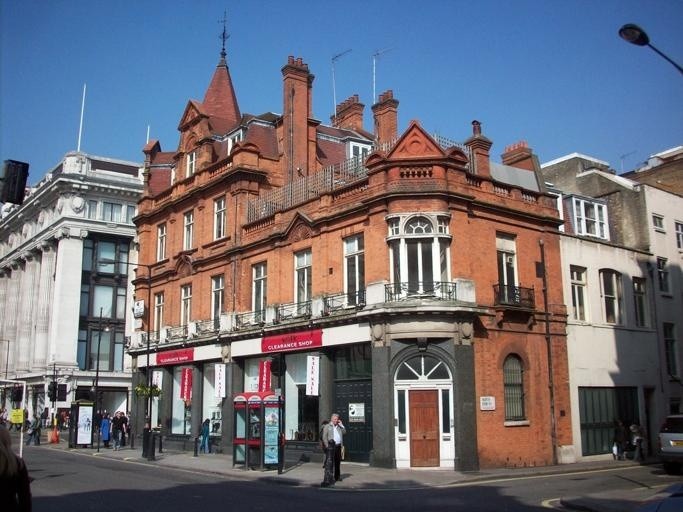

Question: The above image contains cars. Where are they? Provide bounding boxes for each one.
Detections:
[633,481,682,512]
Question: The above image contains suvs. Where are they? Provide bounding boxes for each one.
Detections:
[655,412,683,470]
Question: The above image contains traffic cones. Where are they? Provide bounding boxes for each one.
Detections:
[51,424,60,444]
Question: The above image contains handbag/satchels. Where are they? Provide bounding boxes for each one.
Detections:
[341,446,345,460]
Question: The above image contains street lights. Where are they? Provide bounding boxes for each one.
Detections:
[84,306,114,412]
[616,18,683,75]
[92,255,153,461]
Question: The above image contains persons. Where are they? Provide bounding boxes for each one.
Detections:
[612,420,630,462]
[196,418,209,454]
[94,408,130,451]
[0,407,66,447]
[320,413,346,483]
[0,422,32,511]
[318,421,330,469]
[628,422,647,462]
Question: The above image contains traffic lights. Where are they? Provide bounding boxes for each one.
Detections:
[96,389,104,403]
[47,381,57,402]
